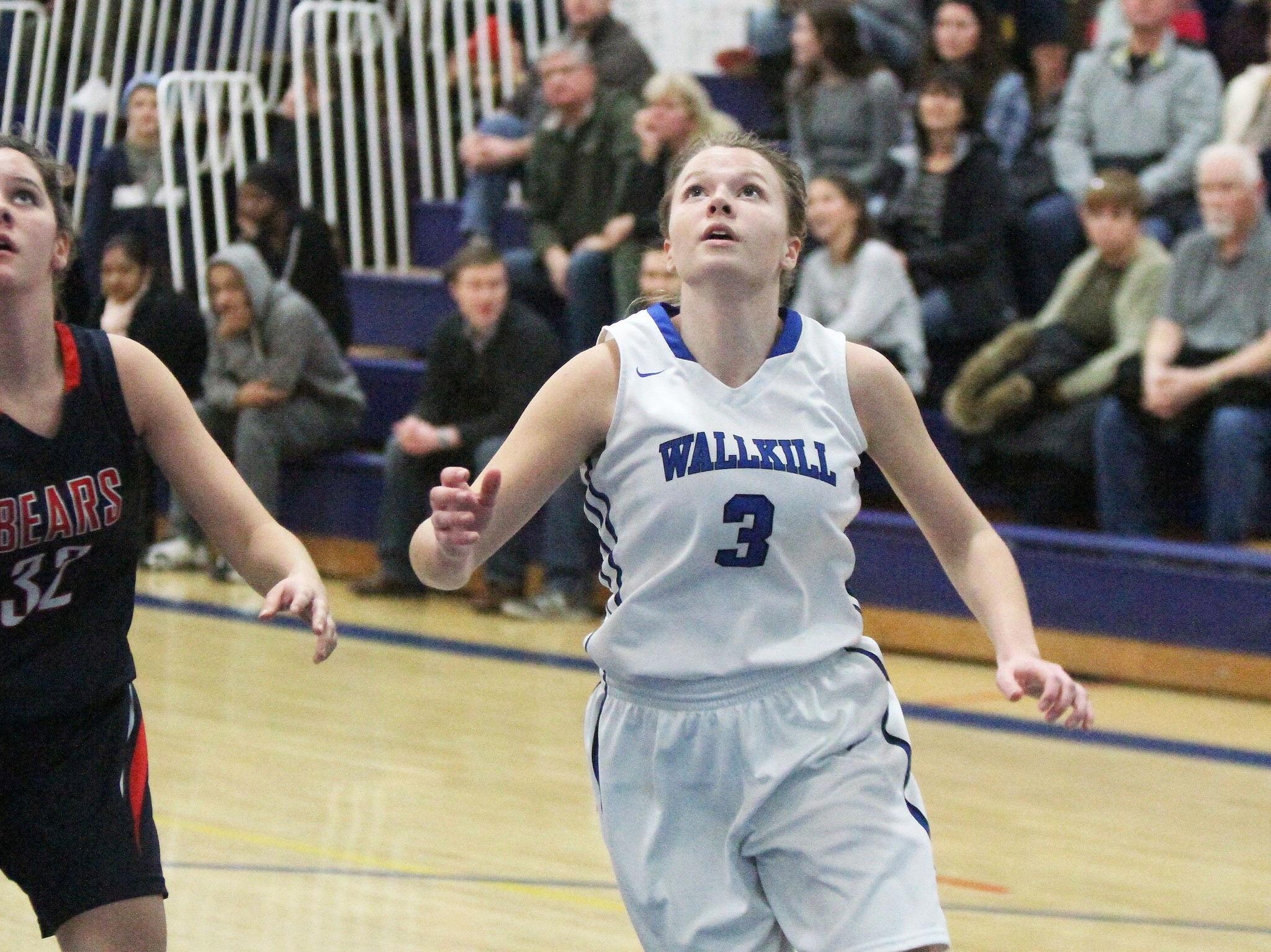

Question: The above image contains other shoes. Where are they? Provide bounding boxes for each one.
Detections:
[351,572,429,599]
[144,538,207,569]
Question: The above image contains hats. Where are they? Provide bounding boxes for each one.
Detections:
[121,71,161,102]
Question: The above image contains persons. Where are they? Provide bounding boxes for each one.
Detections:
[0,138,338,952]
[407,129,1096,952]
[0,0,1271,620]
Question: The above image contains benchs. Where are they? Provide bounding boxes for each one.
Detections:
[277,201,1271,660]
